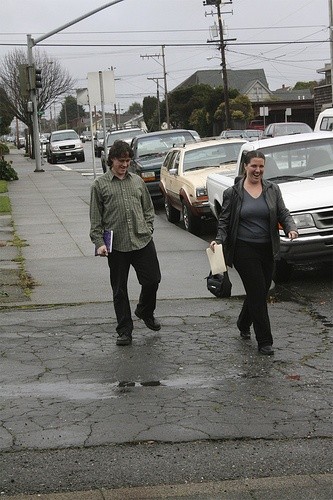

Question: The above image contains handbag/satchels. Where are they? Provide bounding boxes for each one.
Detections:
[204,240,232,298]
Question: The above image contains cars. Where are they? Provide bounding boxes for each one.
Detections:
[0,107,333,166]
[100,127,151,173]
[128,129,203,201]
[46,129,86,165]
[157,137,250,235]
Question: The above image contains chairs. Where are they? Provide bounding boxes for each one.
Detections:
[262,157,280,178]
[309,150,331,170]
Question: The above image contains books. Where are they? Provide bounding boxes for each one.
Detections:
[95,230,113,257]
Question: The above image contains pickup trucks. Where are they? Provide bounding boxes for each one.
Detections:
[205,130,333,281]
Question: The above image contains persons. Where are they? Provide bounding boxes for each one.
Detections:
[89,140,162,345]
[210,150,299,355]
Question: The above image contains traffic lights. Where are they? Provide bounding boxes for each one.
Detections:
[29,68,42,90]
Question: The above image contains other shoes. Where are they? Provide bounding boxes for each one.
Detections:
[259,345,275,355]
[116,324,134,345]
[240,327,251,338]
[134,307,161,331]
